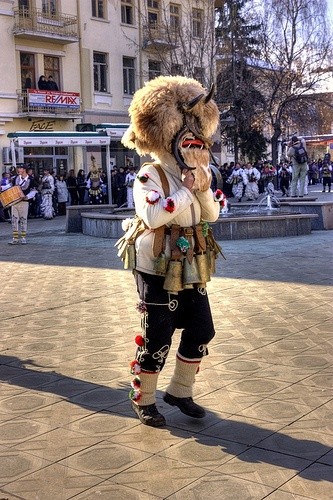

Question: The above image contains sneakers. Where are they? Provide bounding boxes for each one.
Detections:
[8,239,18,244]
[130,399,164,425]
[22,238,26,244]
[164,391,207,418]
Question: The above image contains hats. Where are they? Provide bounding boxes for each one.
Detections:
[18,164,27,169]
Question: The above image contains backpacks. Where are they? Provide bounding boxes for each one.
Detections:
[292,143,308,163]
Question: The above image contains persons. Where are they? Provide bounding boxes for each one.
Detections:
[120,76,221,427]
[208,136,333,214]
[37,75,61,112]
[0,164,137,244]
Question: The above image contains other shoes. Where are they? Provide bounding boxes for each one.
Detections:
[282,193,286,196]
[246,198,252,201]
